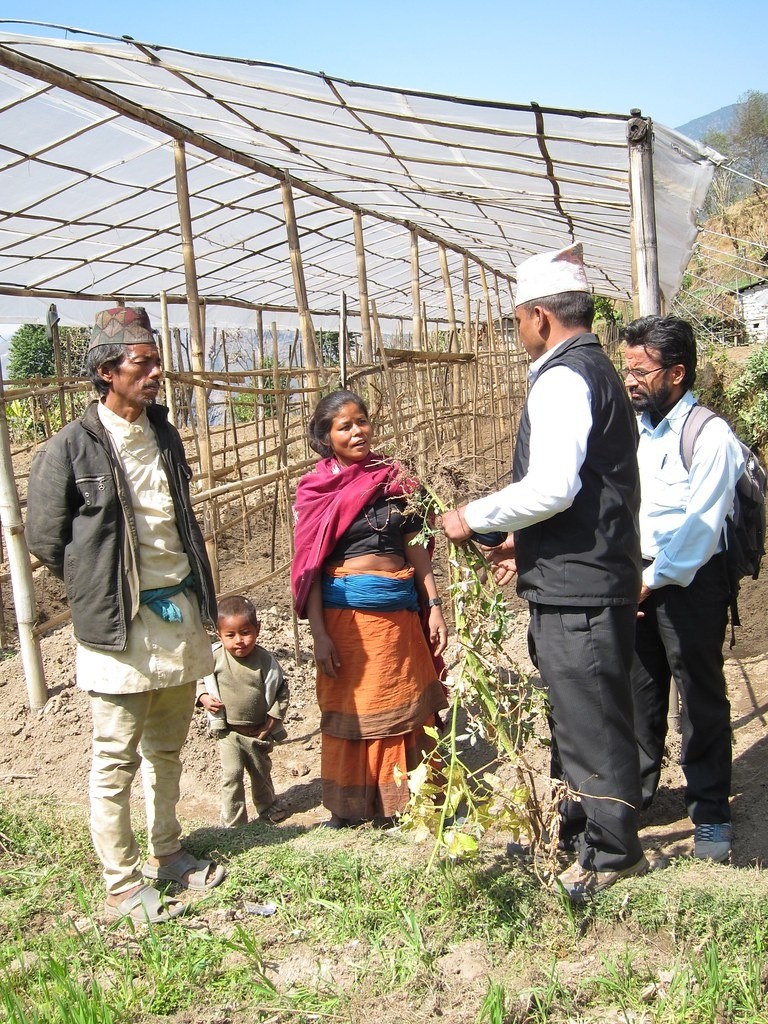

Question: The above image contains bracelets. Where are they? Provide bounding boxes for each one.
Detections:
[425,598,443,608]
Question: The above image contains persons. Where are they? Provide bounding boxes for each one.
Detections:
[25,308,226,922]
[617,313,745,861]
[292,389,449,832]
[194,595,290,826]
[441,241,653,897]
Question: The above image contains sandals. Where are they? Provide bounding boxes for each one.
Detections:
[261,802,287,823]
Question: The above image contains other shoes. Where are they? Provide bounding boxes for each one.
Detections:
[443,813,471,829]
[323,819,356,831]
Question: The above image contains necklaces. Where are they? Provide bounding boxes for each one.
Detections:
[364,496,390,531]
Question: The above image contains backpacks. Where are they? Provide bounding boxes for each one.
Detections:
[681,402,767,581]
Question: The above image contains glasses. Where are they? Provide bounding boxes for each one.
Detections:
[617,361,679,382]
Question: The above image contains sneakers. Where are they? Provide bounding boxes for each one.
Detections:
[550,852,650,899]
[507,834,578,864]
[694,819,732,862]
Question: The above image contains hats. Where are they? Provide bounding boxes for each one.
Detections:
[88,307,156,353]
[514,240,591,309]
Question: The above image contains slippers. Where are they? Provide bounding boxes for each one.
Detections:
[142,851,225,890]
[105,885,191,923]
